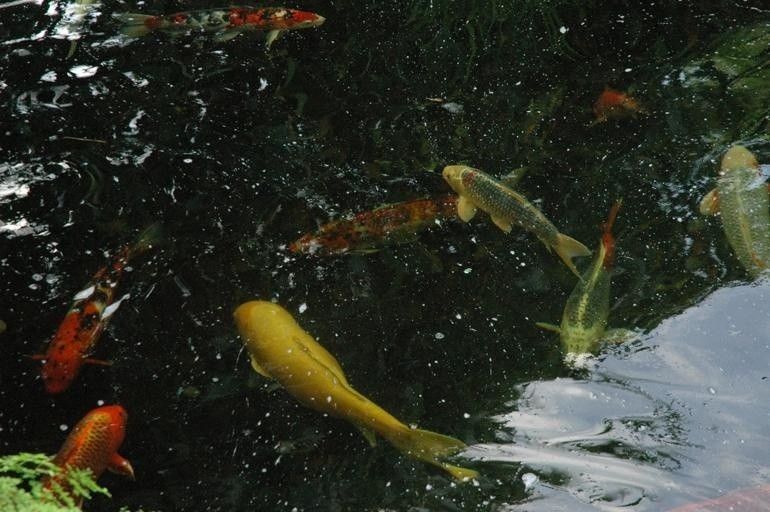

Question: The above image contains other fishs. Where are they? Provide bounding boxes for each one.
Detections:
[664,484,770,511]
[286,195,462,257]
[533,199,641,376]
[700,145,770,288]
[442,164,592,285]
[118,6,328,49]
[40,405,136,512]
[586,84,642,128]
[232,300,480,482]
[30,223,162,394]
